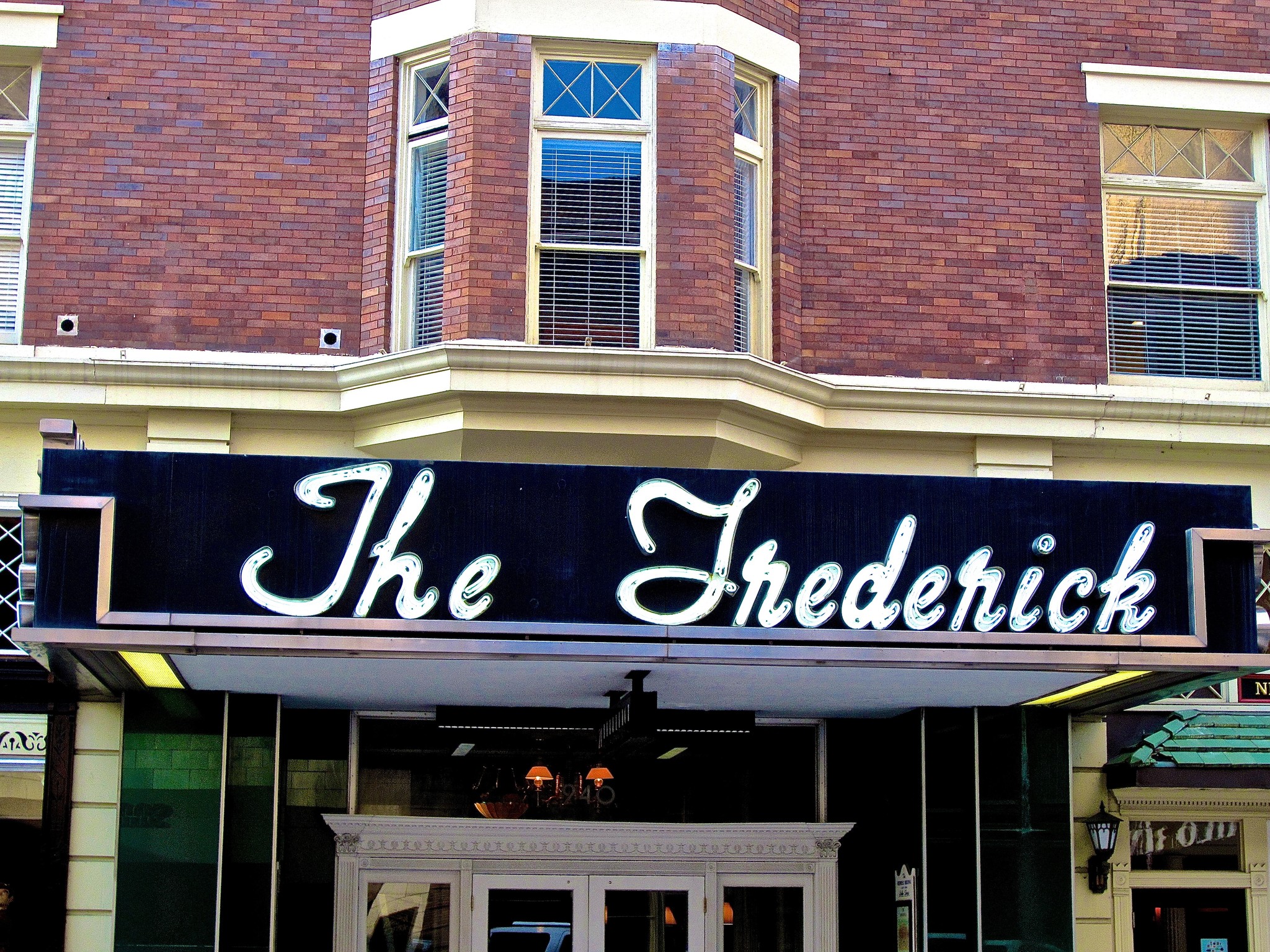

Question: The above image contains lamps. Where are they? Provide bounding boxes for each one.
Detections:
[1085,798,1124,894]
[472,762,615,818]
